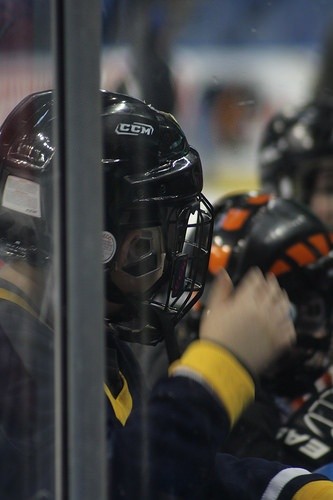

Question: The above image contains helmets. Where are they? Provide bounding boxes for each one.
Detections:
[259,99,333,233]
[2,91,208,303]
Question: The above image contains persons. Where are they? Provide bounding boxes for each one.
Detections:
[182,99,333,484]
[0,88,332,500]
